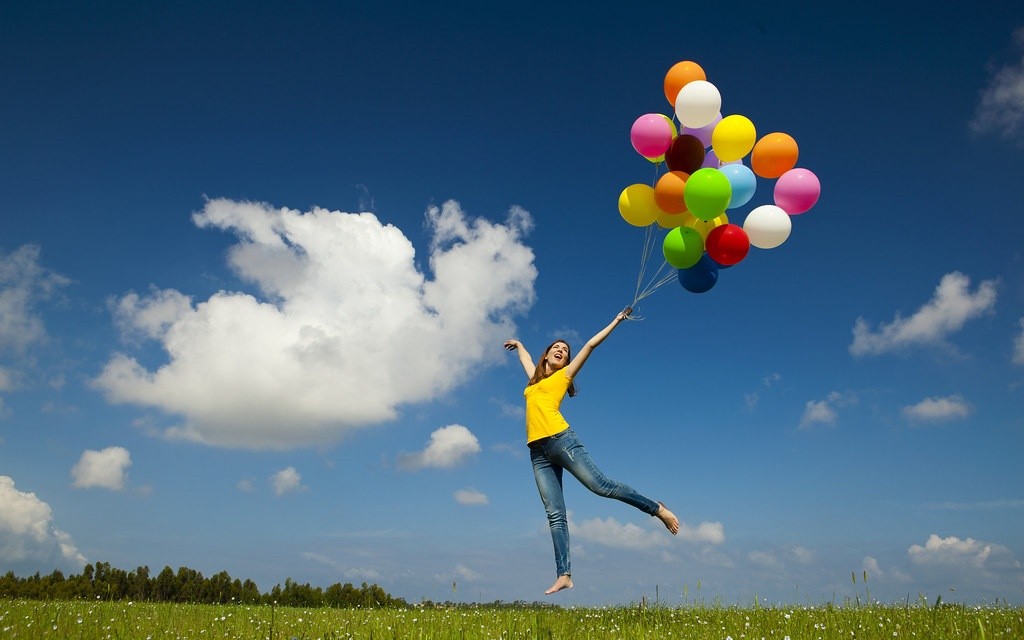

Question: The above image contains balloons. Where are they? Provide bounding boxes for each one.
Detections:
[616,58,825,295]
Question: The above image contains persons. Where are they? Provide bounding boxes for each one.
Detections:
[502,304,680,595]
